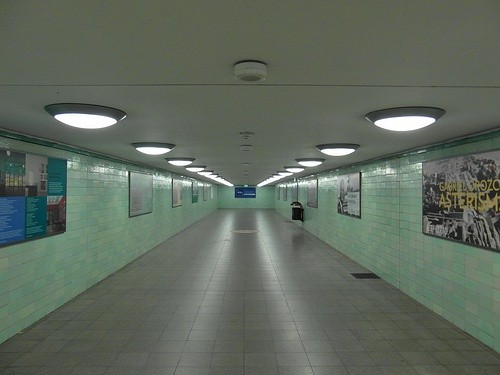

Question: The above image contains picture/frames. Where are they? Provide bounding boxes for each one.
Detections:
[337,171,361,219]
[422,148,500,253]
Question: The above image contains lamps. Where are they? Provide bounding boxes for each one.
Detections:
[285,166,305,173]
[130,143,177,155]
[165,158,196,167]
[43,104,127,129]
[315,144,360,156]
[256,172,293,187]
[295,158,325,167]
[365,107,446,132]
[185,166,235,187]
[232,62,268,83]
